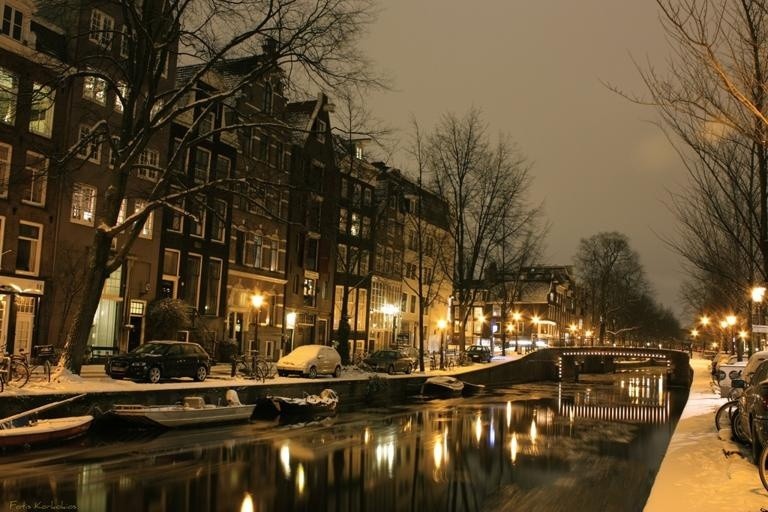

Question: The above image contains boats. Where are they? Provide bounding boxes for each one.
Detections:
[109,390,258,432]
[418,372,468,398]
[264,385,340,423]
[613,358,655,367]
[460,380,485,398]
[0,393,97,454]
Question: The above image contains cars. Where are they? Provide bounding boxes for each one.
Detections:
[702,344,767,468]
[360,349,414,376]
[275,343,342,379]
[463,344,491,364]
[103,338,213,384]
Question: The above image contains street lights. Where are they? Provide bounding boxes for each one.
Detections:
[510,308,525,353]
[478,314,484,345]
[436,319,447,370]
[751,286,764,351]
[531,315,537,338]
[726,315,735,354]
[250,290,261,379]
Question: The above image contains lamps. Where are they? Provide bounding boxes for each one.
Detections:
[139,282,152,298]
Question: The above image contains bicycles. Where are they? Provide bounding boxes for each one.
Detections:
[0,340,54,391]
[233,353,269,378]
